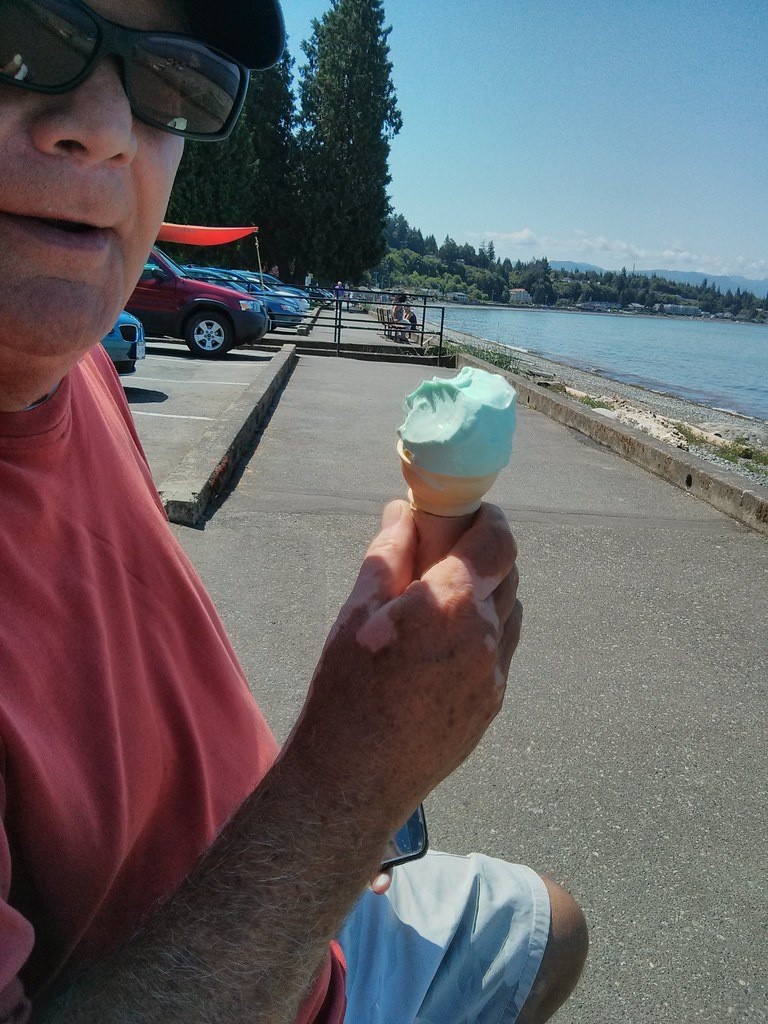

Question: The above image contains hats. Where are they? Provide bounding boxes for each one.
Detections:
[186,1,286,70]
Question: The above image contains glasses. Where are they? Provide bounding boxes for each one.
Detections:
[0,0,250,143]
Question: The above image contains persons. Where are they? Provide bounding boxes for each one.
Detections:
[332,281,344,312]
[389,299,403,322]
[0,0,586,1024]
[401,305,417,332]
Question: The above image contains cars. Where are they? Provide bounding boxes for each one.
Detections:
[99,309,150,380]
[143,259,338,328]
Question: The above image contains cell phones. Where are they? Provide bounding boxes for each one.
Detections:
[379,801,427,872]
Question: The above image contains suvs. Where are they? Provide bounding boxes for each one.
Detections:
[120,243,272,358]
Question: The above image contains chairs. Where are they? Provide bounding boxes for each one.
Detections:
[377,307,411,345]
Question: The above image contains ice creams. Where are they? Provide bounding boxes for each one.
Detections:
[396,365,519,579]
